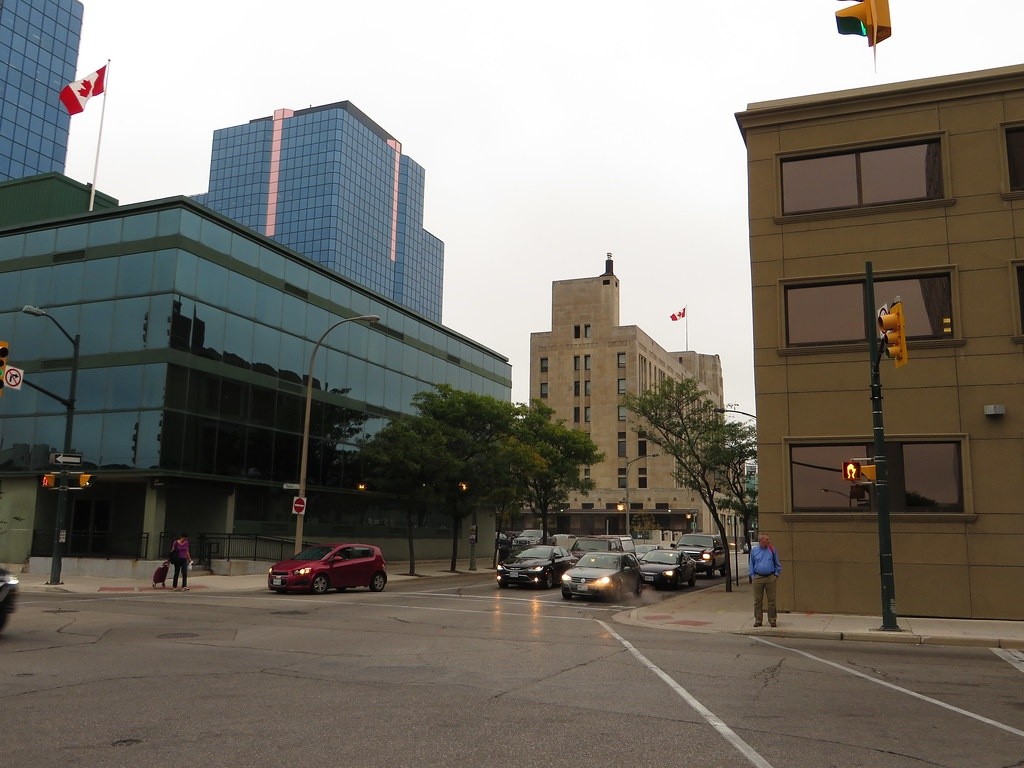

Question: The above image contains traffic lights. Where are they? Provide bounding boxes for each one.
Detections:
[861,465,876,481]
[835,0,891,47]
[38,472,56,488]
[0,341,9,399]
[79,474,98,488]
[841,461,862,481]
[877,301,908,369]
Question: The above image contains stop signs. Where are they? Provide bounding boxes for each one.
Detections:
[291,497,308,515]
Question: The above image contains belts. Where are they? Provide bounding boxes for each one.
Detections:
[753,571,774,578]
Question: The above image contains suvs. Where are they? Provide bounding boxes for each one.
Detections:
[669,533,727,578]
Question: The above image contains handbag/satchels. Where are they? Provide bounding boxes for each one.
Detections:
[187,561,193,571]
[170,539,179,565]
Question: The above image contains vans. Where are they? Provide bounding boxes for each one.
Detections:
[572,535,636,562]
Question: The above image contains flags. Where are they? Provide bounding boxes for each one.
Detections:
[60,60,108,115]
[670,308,685,321]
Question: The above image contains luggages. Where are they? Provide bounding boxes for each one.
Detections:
[151,561,169,588]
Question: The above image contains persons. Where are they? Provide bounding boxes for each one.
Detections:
[749,532,781,627]
[168,533,193,591]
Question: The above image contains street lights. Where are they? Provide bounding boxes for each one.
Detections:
[21,305,81,585]
[625,452,661,534]
[294,314,380,555]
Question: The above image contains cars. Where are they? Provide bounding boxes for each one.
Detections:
[562,552,646,601]
[267,542,388,593]
[635,544,666,560]
[639,549,699,589]
[496,545,579,591]
[470,528,577,550]
[0,566,19,631]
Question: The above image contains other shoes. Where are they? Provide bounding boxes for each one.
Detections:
[182,587,189,591]
[172,588,178,591]
[770,622,775,626]
[753,622,763,627]
